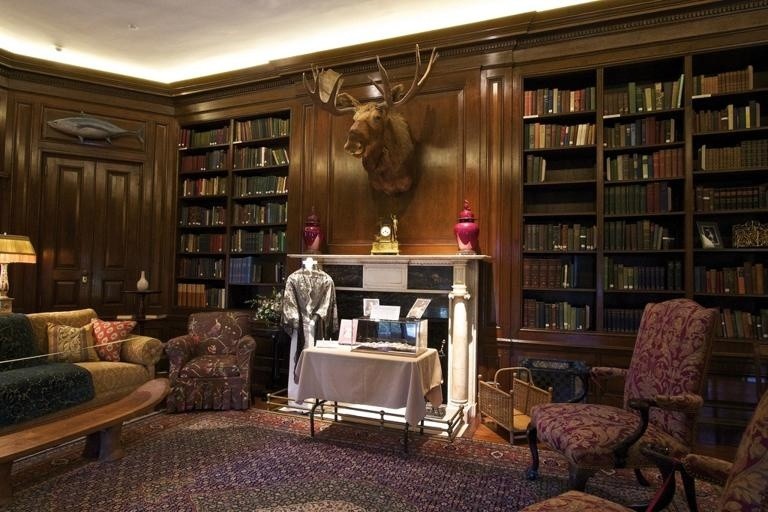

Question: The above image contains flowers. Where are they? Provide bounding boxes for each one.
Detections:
[245,283,286,328]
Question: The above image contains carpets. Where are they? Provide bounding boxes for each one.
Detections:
[0,403,726,512]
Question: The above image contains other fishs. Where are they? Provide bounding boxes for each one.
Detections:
[46,117,146,146]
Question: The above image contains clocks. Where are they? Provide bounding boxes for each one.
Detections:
[367,210,403,255]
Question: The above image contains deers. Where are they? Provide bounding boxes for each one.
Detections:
[302,43,440,196]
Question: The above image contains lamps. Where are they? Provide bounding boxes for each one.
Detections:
[0,231,41,314]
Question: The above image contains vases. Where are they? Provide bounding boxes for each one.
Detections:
[268,316,281,330]
[134,270,152,292]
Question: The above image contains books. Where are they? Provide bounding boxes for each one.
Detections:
[117,312,134,319]
[523,224,597,333]
[524,87,597,187]
[603,74,685,180]
[176,117,288,308]
[144,312,167,318]
[693,66,766,169]
[694,184,766,340]
[604,184,684,334]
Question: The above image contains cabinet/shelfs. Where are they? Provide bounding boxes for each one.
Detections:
[512,24,768,343]
[168,98,291,310]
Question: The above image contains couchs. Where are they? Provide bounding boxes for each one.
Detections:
[1,306,166,416]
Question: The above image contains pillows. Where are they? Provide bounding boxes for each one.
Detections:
[90,315,137,364]
[45,320,102,364]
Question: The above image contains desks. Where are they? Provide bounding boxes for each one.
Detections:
[247,318,293,403]
[98,289,169,337]
[288,342,447,457]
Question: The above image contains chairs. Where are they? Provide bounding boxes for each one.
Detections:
[160,307,260,418]
[519,296,722,511]
[506,385,768,511]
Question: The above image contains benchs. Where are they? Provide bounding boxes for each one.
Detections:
[0,375,172,499]
